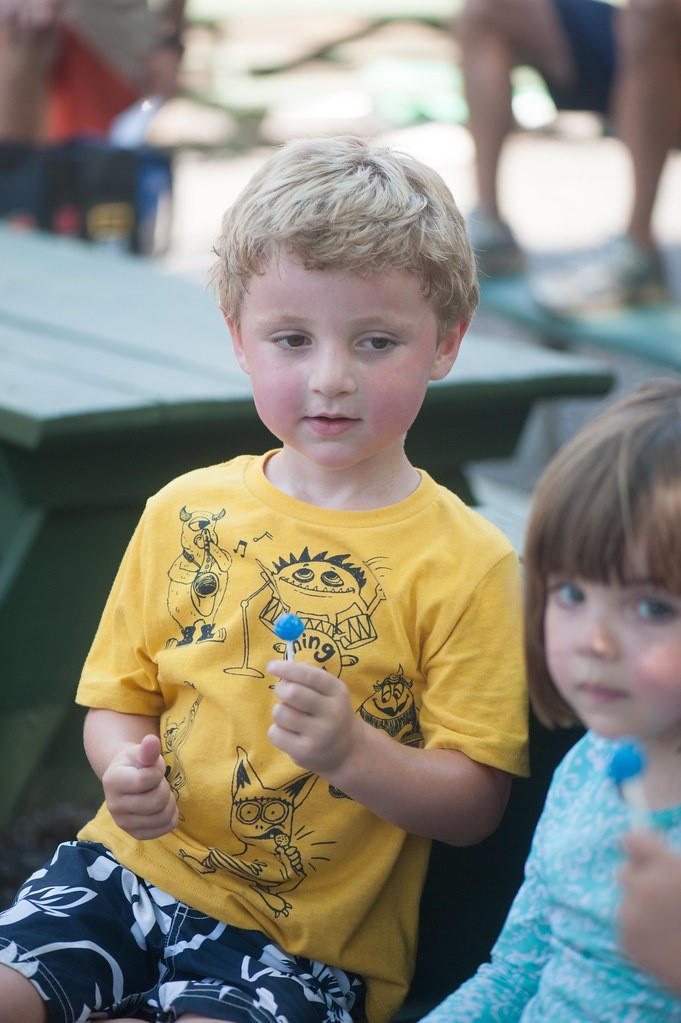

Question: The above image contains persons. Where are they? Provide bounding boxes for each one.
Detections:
[0,135,532,1023]
[411,378,681,1023]
[0,0,186,258]
[449,0,680,315]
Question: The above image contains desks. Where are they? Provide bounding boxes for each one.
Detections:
[0,220,618,902]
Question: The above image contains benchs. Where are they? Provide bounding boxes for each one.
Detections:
[472,247,681,462]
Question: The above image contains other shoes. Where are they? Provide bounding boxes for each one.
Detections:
[445,229,520,286]
[529,239,667,321]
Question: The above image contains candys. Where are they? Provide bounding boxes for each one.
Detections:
[605,740,648,837]
[274,615,303,677]
